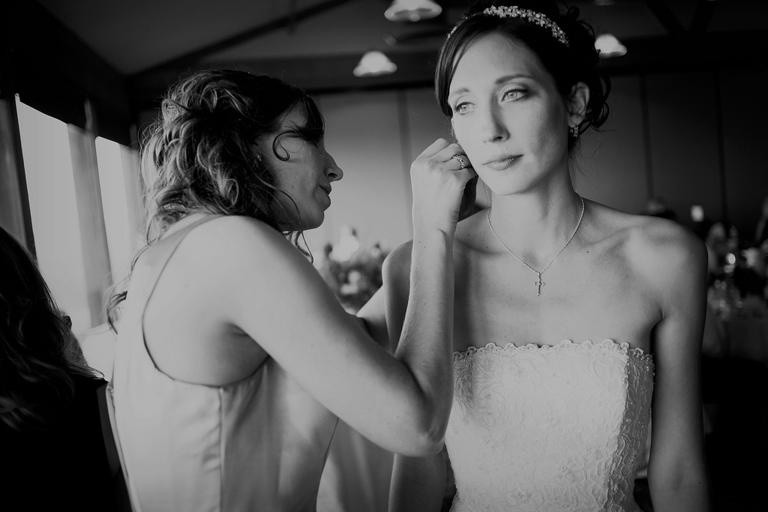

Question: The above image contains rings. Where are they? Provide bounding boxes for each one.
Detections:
[452,154,466,168]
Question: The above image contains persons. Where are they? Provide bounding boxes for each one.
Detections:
[317,228,389,304]
[105,68,478,512]
[381,0,711,512]
[639,194,768,300]
[0,227,108,428]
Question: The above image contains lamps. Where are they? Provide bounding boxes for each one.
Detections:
[352,0,628,78]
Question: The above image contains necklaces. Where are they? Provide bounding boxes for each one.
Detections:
[486,193,585,296]
